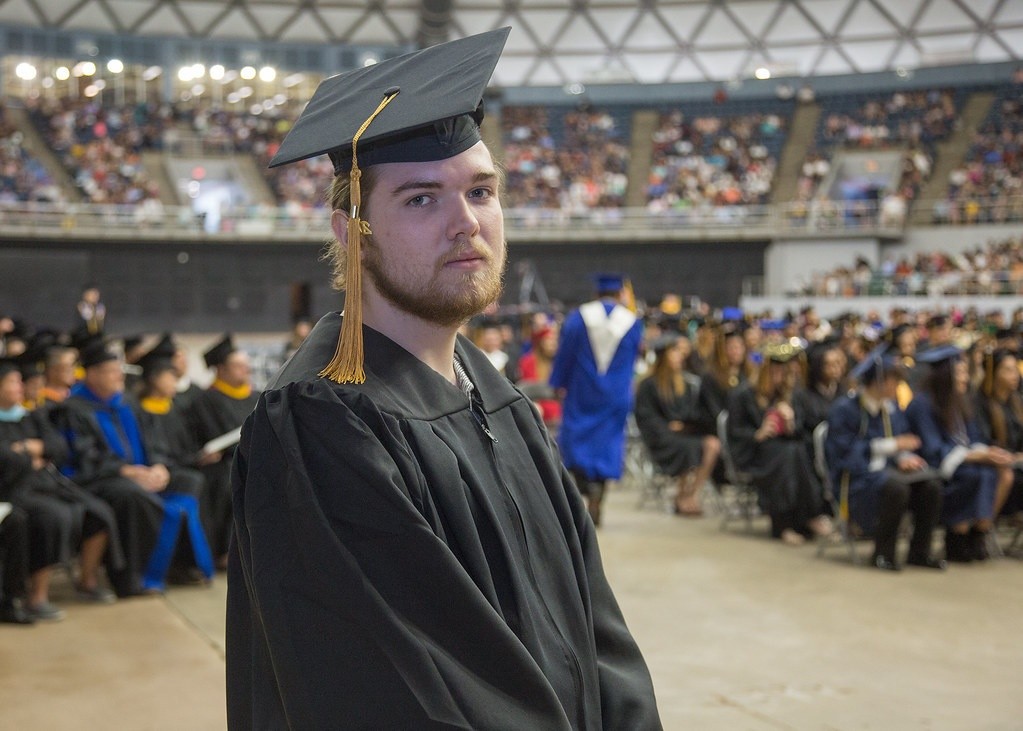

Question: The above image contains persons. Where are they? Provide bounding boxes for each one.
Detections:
[542,271,648,528]
[0,90,1023,227]
[0,237,1023,626]
[225,26,665,731]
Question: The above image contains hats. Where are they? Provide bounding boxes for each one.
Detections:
[75,335,117,369]
[268,25,511,383]
[916,345,961,369]
[848,344,890,380]
[595,274,623,290]
[0,325,70,381]
[134,335,174,372]
[204,331,236,368]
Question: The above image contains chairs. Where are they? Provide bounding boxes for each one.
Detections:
[619,409,1007,562]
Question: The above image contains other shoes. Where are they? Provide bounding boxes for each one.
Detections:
[674,494,704,516]
[906,536,941,568]
[72,584,116,603]
[873,536,901,570]
[946,527,989,561]
[24,602,65,620]
[0,601,36,624]
